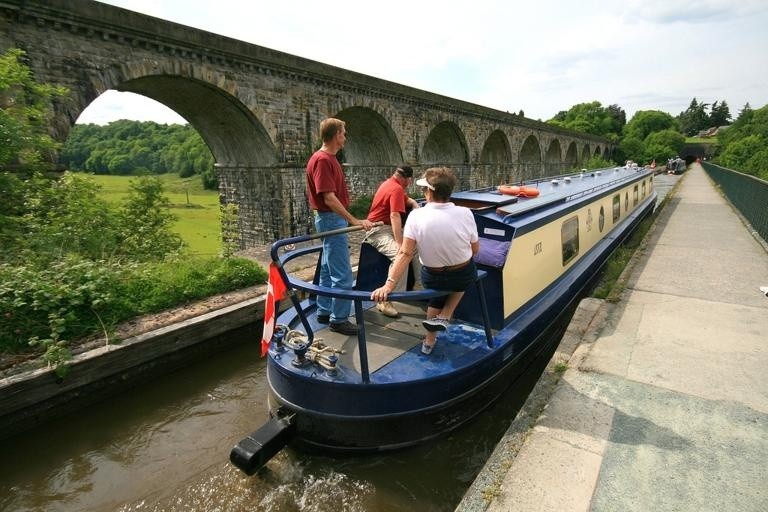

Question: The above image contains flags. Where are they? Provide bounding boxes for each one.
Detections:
[257,265,289,357]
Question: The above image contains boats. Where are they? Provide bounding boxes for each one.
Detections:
[665,155,687,175]
[229,163,657,476]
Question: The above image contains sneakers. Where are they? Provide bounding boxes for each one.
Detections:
[421,314,451,333]
[327,319,364,336]
[375,299,399,319]
[421,336,437,355]
[316,313,330,324]
[412,281,425,291]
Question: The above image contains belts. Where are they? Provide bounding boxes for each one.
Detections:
[421,257,475,273]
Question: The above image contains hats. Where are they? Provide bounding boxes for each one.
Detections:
[395,166,415,178]
[414,175,441,193]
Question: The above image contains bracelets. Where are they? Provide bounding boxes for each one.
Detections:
[385,276,396,284]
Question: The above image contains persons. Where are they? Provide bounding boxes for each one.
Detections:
[667,153,712,171]
[363,164,430,317]
[370,166,480,357]
[304,117,375,336]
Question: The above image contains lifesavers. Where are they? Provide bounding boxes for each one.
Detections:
[497,183,540,197]
